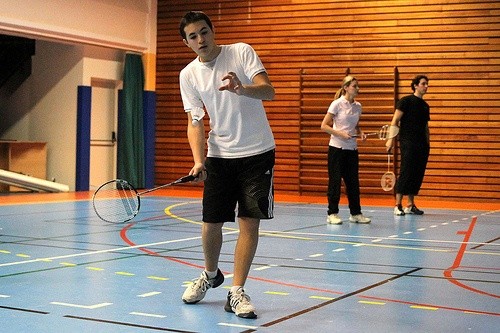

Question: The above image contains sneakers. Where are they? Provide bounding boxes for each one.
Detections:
[348,214,371,223]
[394,205,406,215]
[224,290,258,319]
[326,214,342,224]
[183,268,224,304]
[404,204,424,215]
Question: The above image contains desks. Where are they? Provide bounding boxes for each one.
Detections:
[0,140,47,192]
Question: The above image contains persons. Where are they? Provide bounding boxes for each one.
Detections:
[320,75,372,226]
[180,9,276,319]
[384,75,431,214]
